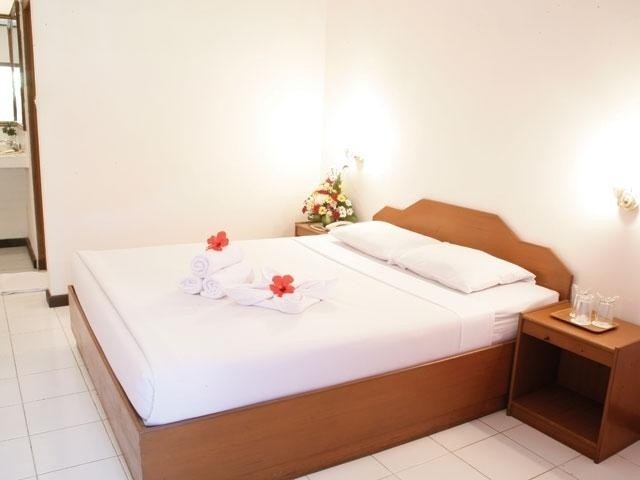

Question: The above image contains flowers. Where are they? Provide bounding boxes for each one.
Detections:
[299,153,356,225]
[204,230,231,252]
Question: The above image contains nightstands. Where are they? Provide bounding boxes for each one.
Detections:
[293,221,341,236]
[507,304,640,465]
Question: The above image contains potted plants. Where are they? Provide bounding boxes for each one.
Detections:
[0,121,20,152]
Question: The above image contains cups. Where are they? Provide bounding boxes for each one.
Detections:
[571,284,618,328]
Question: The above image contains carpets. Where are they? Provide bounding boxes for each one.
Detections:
[0,271,46,293]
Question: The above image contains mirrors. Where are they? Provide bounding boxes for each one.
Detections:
[0,0,27,130]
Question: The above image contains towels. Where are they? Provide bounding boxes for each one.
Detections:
[181,246,321,315]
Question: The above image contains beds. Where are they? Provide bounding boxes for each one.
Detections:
[67,198,572,479]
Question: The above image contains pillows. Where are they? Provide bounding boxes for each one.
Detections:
[329,220,446,264]
[395,239,536,292]
